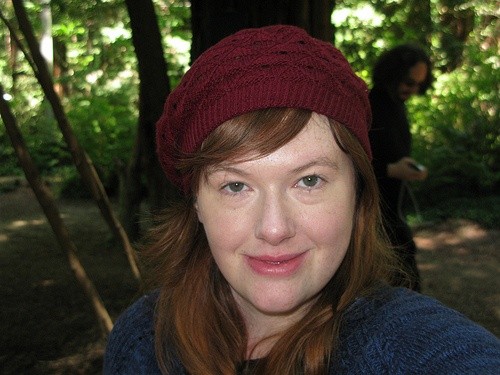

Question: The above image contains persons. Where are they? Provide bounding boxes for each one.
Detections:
[369,44,433,295]
[103,25,500,375]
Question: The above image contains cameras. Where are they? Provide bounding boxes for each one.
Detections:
[408,162,425,172]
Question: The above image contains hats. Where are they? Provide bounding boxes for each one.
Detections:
[155,24,373,196]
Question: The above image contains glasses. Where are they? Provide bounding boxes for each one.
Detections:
[402,78,427,88]
